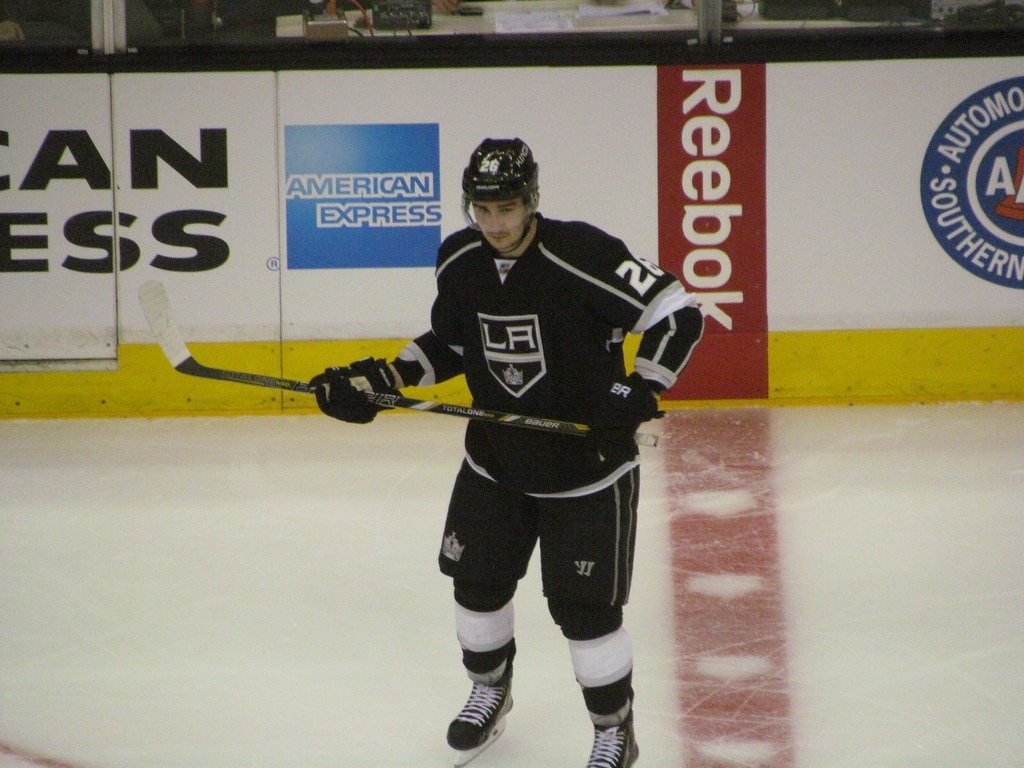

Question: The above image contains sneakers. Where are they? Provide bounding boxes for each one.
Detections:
[576,668,638,768]
[447,638,517,765]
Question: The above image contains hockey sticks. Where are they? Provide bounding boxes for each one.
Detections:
[136,279,659,448]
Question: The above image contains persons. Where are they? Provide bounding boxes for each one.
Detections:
[311,138,706,768]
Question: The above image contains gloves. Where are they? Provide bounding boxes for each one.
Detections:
[573,371,665,458]
[308,356,404,424]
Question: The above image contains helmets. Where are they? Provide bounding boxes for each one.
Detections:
[461,137,538,218]
